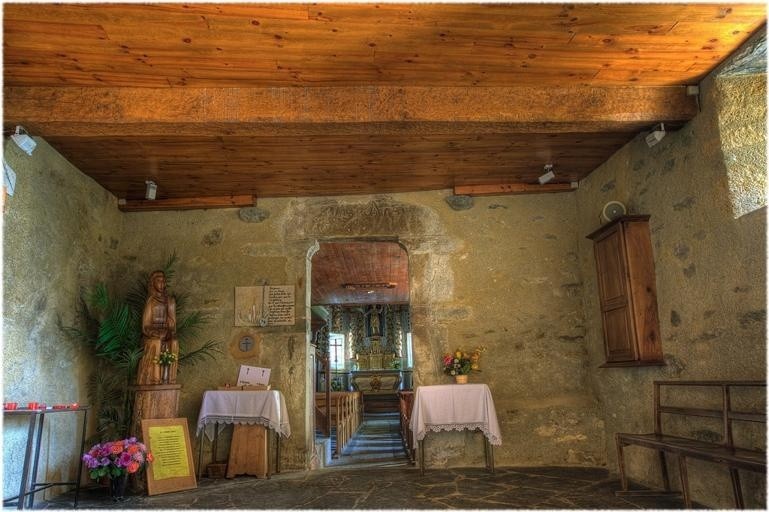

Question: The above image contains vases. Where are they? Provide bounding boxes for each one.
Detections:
[455,375,468,384]
[163,366,169,384]
[110,475,131,501]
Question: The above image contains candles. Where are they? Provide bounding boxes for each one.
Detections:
[4,402,79,410]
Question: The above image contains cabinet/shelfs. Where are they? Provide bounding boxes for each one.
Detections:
[585,214,667,368]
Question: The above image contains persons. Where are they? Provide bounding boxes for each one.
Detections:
[133,269,181,382]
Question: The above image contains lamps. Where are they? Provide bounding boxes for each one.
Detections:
[645,123,666,148]
[10,126,37,157]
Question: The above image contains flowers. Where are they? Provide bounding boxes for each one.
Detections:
[440,351,481,376]
[81,437,154,483]
[153,349,178,368]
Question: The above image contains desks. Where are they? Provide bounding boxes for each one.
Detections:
[409,384,503,477]
[2,408,92,509]
[194,390,291,481]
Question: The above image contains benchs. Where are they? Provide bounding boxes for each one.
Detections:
[399,391,415,465]
[315,391,364,459]
[615,381,766,509]
